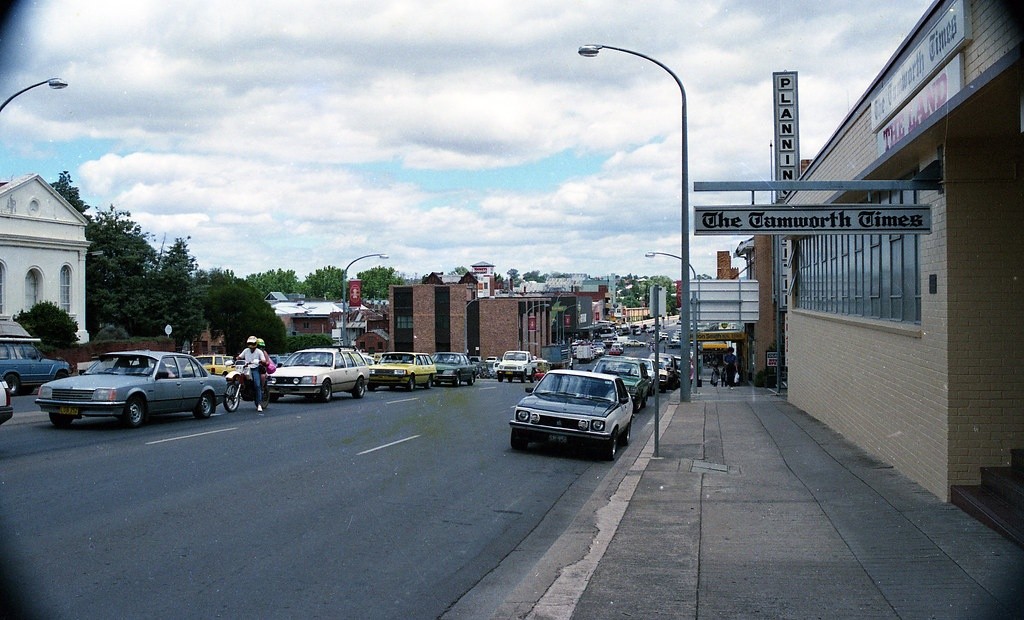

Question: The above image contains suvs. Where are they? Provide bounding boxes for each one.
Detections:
[0,340,72,396]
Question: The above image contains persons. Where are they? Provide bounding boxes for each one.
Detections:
[256,339,270,385]
[236,335,266,412]
[723,347,738,389]
[707,358,727,387]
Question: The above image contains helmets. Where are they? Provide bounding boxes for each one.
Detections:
[256,338,265,346]
[246,336,257,349]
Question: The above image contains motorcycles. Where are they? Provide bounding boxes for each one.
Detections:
[223,354,272,412]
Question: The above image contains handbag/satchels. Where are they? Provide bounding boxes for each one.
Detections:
[263,350,276,374]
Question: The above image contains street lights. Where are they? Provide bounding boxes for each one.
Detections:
[645,251,698,394]
[342,253,389,347]
[577,44,691,404]
[464,296,495,354]
[526,303,550,350]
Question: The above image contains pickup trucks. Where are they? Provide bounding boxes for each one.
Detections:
[497,351,549,383]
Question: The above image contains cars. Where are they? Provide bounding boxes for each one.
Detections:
[540,322,683,391]
[35,350,229,428]
[509,369,634,462]
[184,354,237,377]
[0,374,13,425]
[269,347,370,404]
[360,353,383,365]
[235,353,292,371]
[469,355,500,364]
[585,358,651,413]
[429,352,476,387]
[368,351,437,392]
[618,357,661,396]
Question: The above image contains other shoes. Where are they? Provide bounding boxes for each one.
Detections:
[257,405,262,411]
